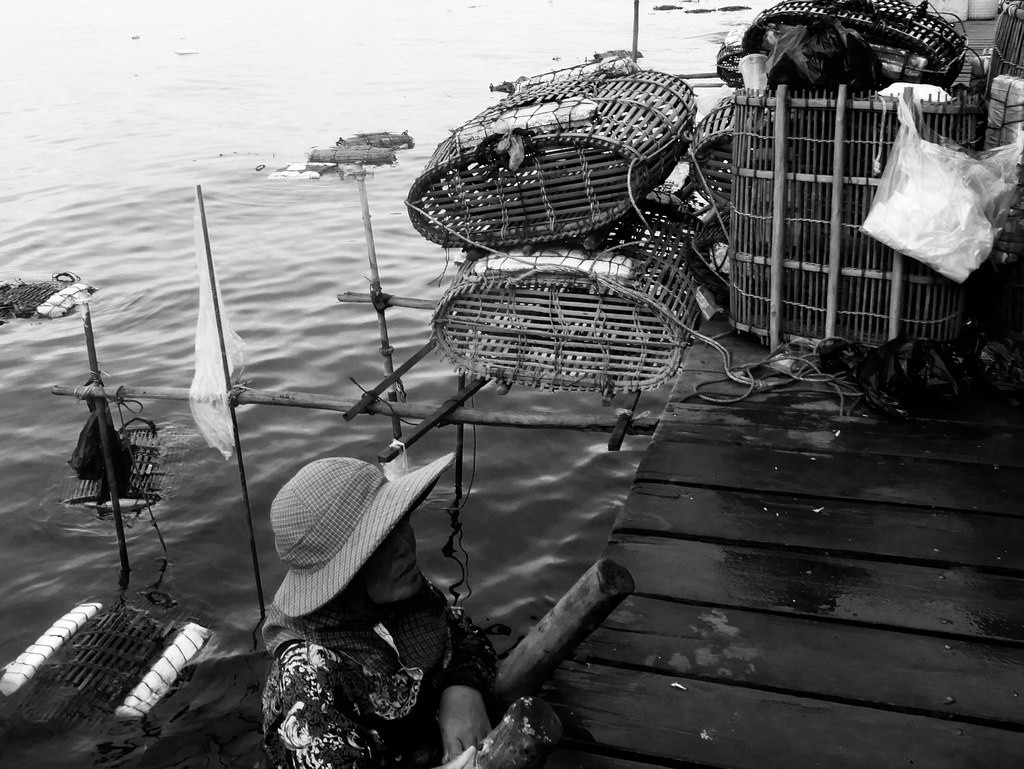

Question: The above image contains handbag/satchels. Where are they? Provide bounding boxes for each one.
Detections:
[859,94,1023,284]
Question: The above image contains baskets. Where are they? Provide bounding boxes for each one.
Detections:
[57,419,187,530]
[405,0,1024,394]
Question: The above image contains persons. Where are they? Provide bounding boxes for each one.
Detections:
[262,453,497,769]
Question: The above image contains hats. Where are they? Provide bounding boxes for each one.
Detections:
[274,452,457,617]
